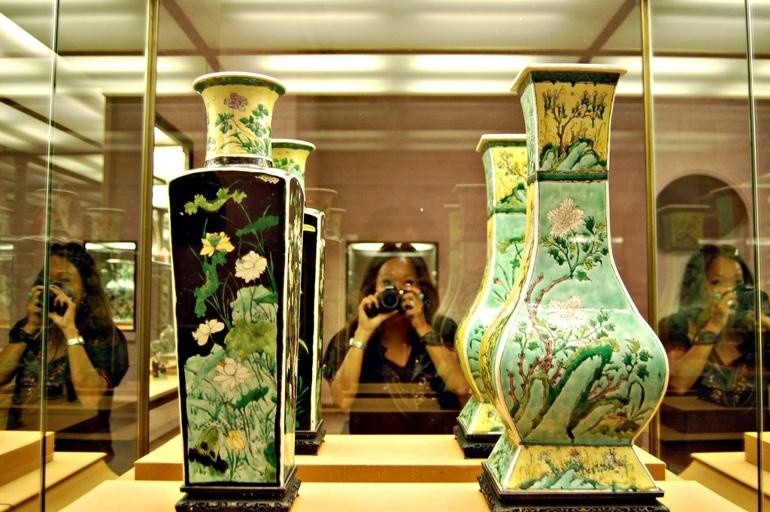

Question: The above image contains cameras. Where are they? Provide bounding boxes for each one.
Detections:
[375,285,407,312]
[733,285,769,310]
[34,280,73,312]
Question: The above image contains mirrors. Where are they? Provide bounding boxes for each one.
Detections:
[0,4,769,511]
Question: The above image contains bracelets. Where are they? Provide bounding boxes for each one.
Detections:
[692,328,719,345]
[9,327,33,346]
[420,329,444,345]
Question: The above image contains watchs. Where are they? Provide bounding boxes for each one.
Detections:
[66,336,85,346]
[349,337,367,350]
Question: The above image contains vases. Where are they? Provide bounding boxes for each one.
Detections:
[475,63,671,511]
[657,184,766,340]
[166,71,302,511]
[304,188,349,378]
[433,182,484,352]
[33,189,126,370]
[452,133,528,459]
[271,138,327,456]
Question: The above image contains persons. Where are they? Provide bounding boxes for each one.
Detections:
[322,241,471,434]
[659,244,770,396]
[0,241,129,463]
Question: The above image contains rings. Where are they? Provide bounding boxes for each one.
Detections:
[715,292,720,300]
[26,291,33,300]
[418,293,424,300]
[727,300,733,306]
